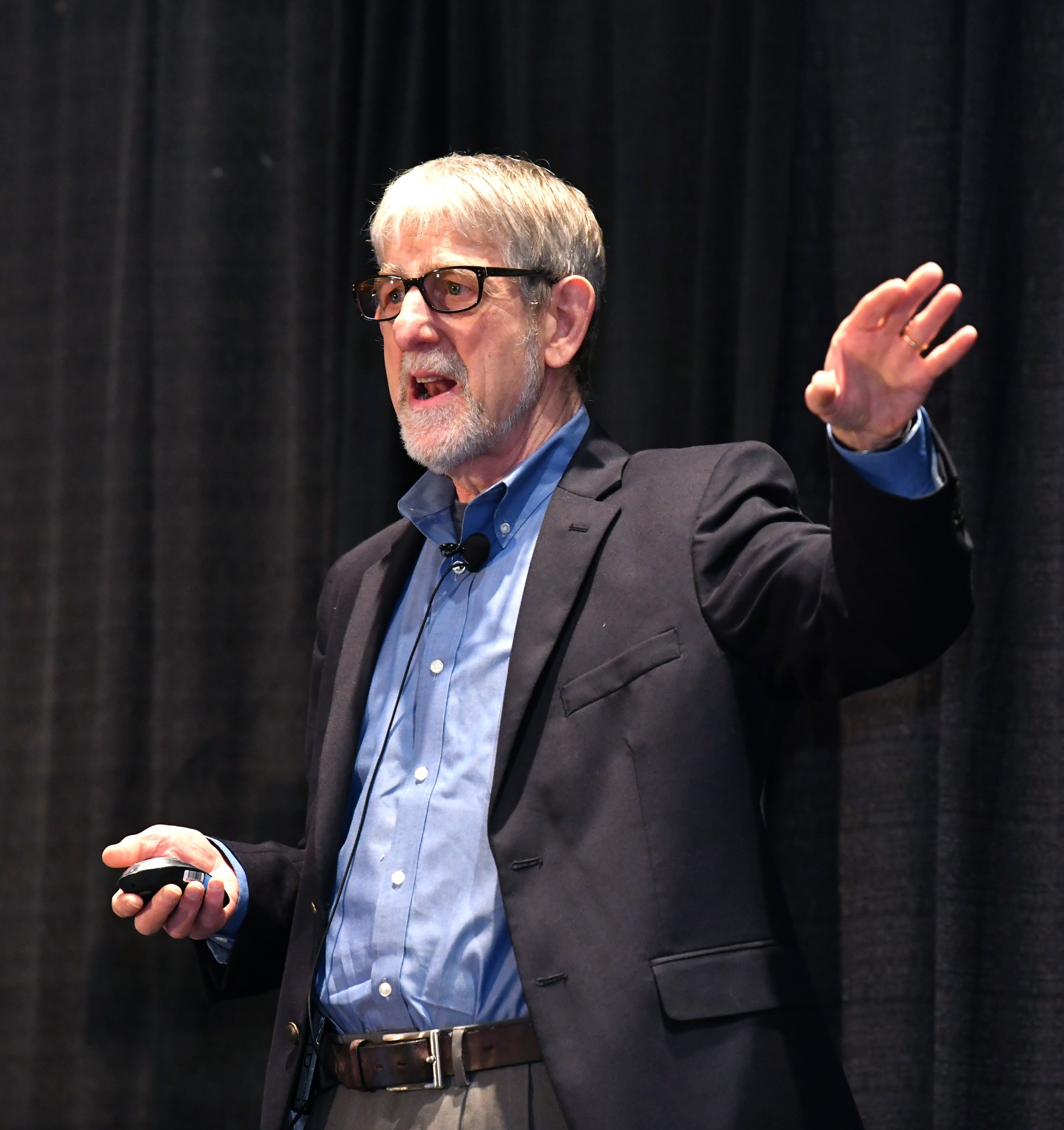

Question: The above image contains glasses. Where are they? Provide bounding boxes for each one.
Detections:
[351,266,559,322]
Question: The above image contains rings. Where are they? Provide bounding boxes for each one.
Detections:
[900,332,930,350]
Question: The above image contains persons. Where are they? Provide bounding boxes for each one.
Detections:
[100,150,988,1129]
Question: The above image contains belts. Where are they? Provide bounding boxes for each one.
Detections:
[332,1017,543,1092]
